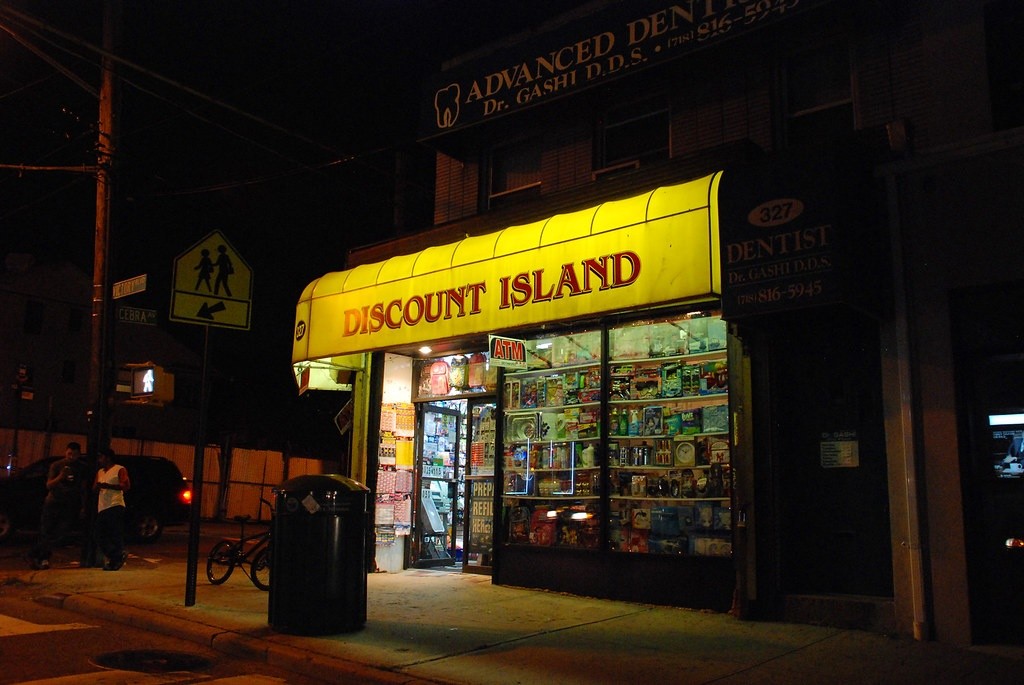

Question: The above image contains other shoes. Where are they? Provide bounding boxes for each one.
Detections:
[103,564,112,571]
[40,560,49,569]
[112,551,127,570]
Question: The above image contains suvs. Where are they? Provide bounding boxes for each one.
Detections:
[1,453,193,545]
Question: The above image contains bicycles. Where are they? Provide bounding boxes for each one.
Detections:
[206,496,276,591]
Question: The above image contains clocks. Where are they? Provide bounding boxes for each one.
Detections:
[674,440,695,466]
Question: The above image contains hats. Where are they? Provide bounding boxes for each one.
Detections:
[98,449,114,458]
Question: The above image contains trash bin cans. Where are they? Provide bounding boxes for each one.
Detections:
[268,474,370,636]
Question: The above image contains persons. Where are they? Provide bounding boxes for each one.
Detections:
[92,449,131,570]
[30,442,87,569]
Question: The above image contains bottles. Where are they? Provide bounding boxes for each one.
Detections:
[542,443,572,469]
[609,408,639,437]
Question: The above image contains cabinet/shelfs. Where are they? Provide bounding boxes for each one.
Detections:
[504,358,733,559]
[418,399,477,551]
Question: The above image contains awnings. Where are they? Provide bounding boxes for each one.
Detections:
[292,169,727,393]
